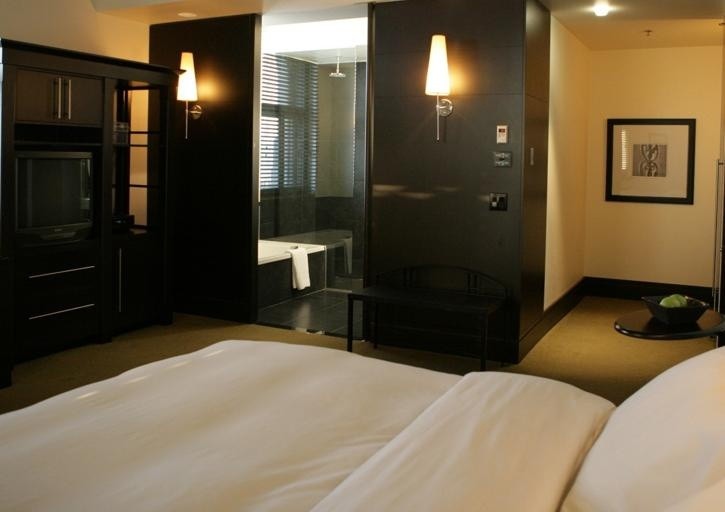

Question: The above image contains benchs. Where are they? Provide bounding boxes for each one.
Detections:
[346,283,504,371]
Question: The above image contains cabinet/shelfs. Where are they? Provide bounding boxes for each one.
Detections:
[0,38,185,394]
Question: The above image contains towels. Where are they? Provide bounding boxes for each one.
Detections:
[285,247,312,291]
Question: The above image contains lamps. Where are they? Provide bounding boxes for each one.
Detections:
[176,52,203,139]
[424,34,454,139]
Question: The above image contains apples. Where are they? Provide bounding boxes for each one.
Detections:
[670,294,688,308]
[660,297,680,307]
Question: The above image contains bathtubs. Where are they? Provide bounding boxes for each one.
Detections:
[257,238,327,310]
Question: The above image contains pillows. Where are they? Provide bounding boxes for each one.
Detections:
[561,342,725,512]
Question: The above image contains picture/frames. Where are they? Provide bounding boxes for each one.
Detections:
[604,118,696,206]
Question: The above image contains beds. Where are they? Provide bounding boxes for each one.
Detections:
[0,338,725,512]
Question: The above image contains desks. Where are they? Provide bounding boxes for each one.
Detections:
[613,308,725,347]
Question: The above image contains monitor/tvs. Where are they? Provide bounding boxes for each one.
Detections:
[14,150,94,247]
